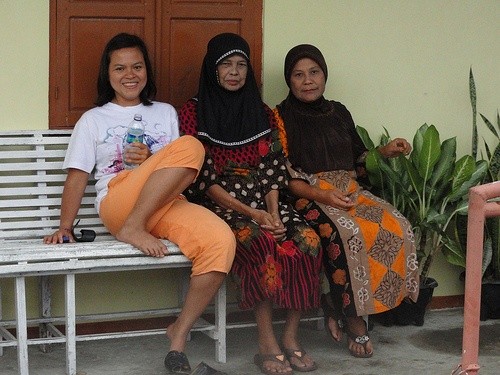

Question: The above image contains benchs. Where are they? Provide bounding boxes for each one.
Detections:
[0,130,325,375]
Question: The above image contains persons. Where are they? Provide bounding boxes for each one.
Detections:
[268,45,421,357]
[177,33,323,375]
[43,33,236,375]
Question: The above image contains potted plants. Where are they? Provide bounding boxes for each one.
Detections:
[356,67,500,326]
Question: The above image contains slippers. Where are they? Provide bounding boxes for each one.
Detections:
[346,320,372,359]
[321,294,342,344]
[254,353,294,375]
[281,345,316,372]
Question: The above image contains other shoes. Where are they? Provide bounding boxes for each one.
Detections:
[165,351,192,375]
[188,362,226,375]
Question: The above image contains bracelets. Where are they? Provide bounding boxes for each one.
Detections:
[60,228,71,232]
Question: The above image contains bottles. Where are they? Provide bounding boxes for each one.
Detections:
[122,114,145,170]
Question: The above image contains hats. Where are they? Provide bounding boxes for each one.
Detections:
[284,44,327,89]
